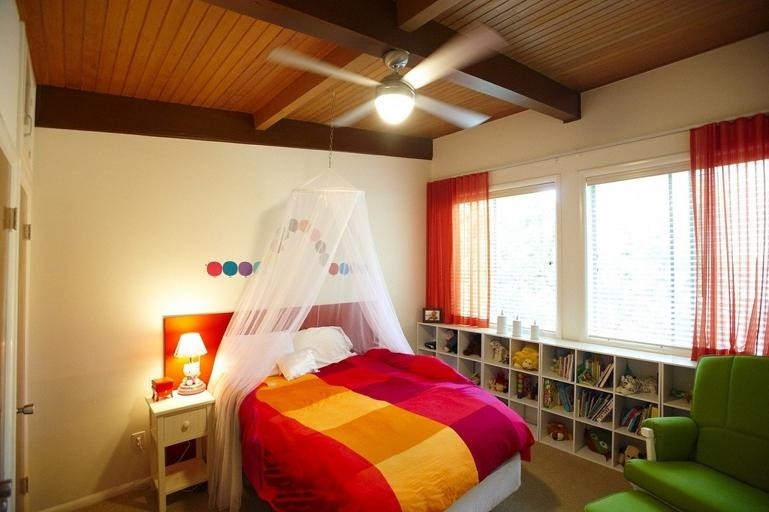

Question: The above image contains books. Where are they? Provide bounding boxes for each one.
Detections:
[516,373,530,399]
[541,351,657,436]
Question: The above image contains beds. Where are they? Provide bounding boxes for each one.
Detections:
[160,299,536,509]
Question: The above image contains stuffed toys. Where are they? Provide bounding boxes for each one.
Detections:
[512,346,538,370]
[443,330,457,355]
[462,334,480,355]
[623,445,641,461]
[582,427,611,460]
[546,422,569,442]
[490,340,509,364]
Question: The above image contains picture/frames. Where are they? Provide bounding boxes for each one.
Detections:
[423,307,441,322]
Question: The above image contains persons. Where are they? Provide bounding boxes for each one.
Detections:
[427,311,438,320]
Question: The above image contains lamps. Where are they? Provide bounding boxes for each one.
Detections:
[174,330,208,395]
[373,67,417,128]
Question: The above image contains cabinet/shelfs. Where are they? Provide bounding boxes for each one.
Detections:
[541,342,698,475]
[416,322,539,449]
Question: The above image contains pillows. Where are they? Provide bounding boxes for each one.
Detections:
[276,347,321,380]
[224,329,294,380]
[295,324,357,375]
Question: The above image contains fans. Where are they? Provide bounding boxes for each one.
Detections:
[254,18,507,135]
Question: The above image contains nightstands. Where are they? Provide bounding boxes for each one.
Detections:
[143,392,219,512]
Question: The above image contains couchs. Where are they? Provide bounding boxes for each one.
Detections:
[583,354,769,512]
